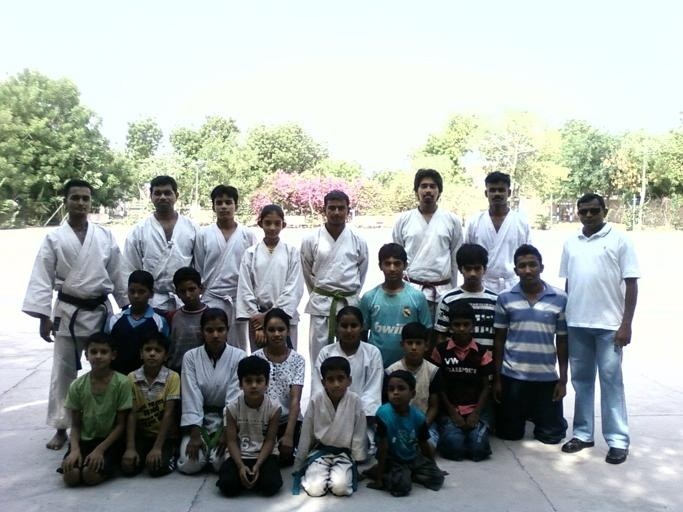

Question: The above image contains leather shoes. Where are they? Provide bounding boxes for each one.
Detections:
[561,435,595,453]
[606,446,629,465]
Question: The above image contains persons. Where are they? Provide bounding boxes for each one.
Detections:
[21,168,642,498]
